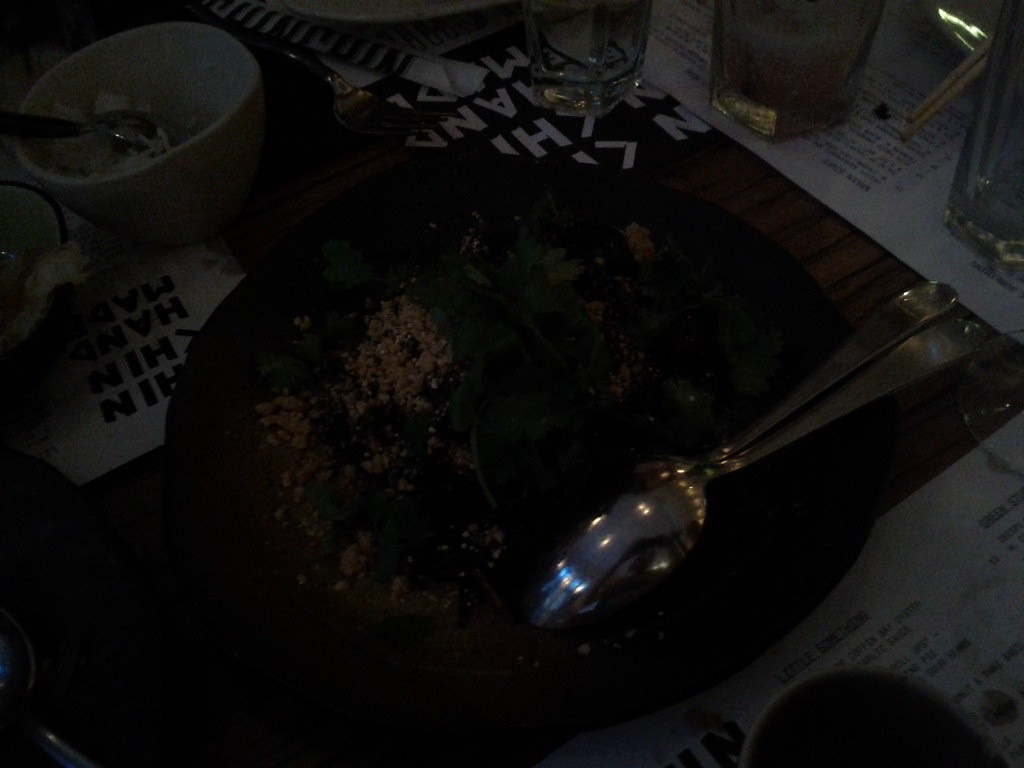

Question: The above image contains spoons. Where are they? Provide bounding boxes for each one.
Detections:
[1,609,103,768]
[519,284,984,626]
[0,110,191,156]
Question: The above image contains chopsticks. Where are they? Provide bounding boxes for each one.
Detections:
[898,35,995,141]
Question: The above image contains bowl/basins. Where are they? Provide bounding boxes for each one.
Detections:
[1,182,71,389]
[738,663,1012,768]
[11,22,265,246]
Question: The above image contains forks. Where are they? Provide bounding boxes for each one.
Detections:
[181,0,459,135]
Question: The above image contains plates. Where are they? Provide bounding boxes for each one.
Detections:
[280,0,511,22]
[166,152,904,718]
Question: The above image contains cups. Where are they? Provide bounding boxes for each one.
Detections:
[522,0,651,115]
[710,0,887,142]
[942,1,1024,298]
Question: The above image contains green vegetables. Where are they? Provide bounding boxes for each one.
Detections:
[257,231,783,569]
